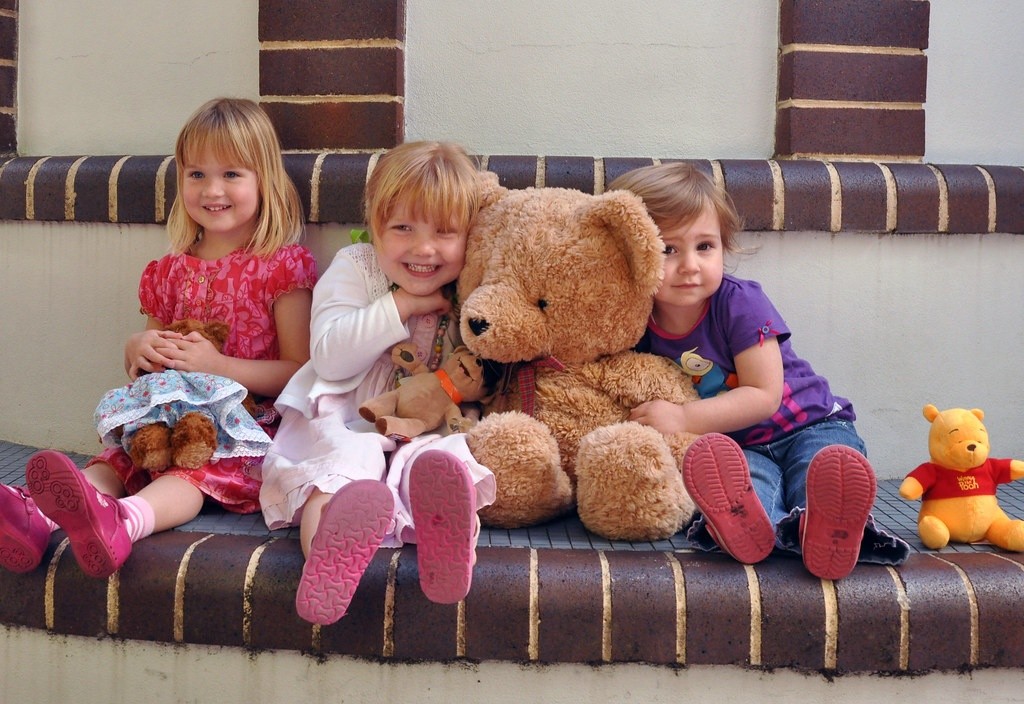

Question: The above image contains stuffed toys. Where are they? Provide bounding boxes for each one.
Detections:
[899,402,1024,554]
[93,317,272,470]
[359,343,495,439]
[455,174,702,542]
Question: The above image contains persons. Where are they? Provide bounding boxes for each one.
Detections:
[603,162,931,578]
[0,98,317,581]
[256,141,496,624]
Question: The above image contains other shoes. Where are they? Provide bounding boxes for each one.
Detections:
[682,432,775,563]
[801,444,877,580]
[408,450,481,605]
[0,481,51,574]
[295,479,394,626]
[26,449,133,579]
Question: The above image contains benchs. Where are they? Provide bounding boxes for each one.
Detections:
[0,441,1024,704]
